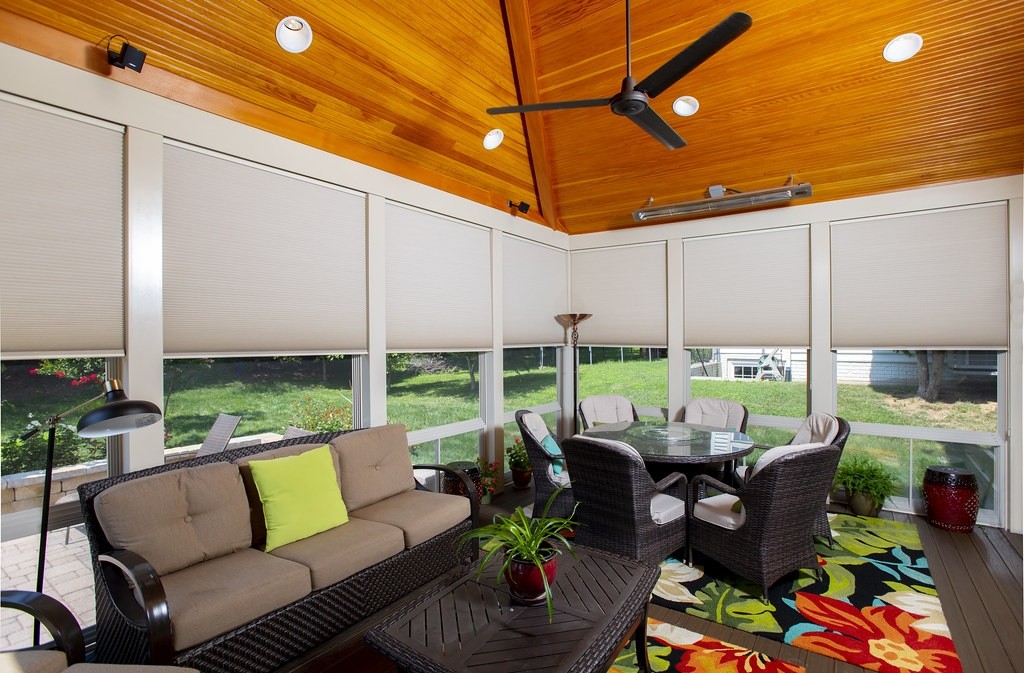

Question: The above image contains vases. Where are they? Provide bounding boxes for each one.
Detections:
[481,490,492,504]
[509,465,532,490]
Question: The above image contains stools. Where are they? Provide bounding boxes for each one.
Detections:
[922,464,980,531]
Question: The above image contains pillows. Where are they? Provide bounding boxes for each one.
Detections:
[541,434,564,475]
[246,444,349,553]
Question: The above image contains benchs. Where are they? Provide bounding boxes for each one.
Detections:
[46,492,84,545]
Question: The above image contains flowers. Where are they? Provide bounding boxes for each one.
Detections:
[475,457,501,496]
[505,437,531,471]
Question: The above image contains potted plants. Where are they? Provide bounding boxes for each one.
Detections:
[452,480,589,625]
[829,454,904,518]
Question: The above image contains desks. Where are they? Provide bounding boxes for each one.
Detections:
[364,538,661,673]
[582,421,755,495]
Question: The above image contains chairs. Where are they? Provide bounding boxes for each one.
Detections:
[195,412,242,458]
[688,441,842,605]
[560,434,688,567]
[667,396,750,488]
[734,410,851,550]
[579,394,639,431]
[1,590,200,673]
[515,410,577,541]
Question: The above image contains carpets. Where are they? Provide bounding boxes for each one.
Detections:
[606,617,806,673]
[649,511,963,673]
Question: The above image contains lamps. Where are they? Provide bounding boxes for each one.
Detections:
[632,183,812,223]
[557,314,592,435]
[18,377,162,648]
[508,201,530,213]
[95,34,148,73]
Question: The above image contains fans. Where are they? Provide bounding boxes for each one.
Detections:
[486,0,752,151]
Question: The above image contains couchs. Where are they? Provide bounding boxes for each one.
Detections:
[76,424,479,673]
